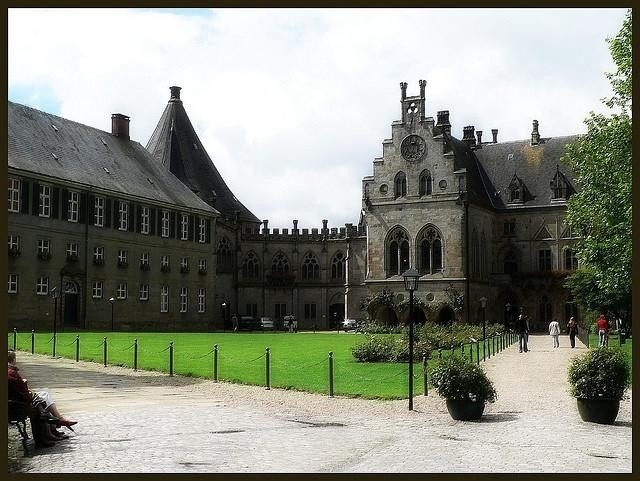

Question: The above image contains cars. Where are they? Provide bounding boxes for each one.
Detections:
[225,314,274,332]
[343,319,356,332]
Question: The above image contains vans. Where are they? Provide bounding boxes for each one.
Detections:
[277,316,297,330]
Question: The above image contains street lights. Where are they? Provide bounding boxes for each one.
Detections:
[506,303,511,332]
[402,268,421,411]
[480,296,488,349]
[334,313,337,331]
[110,297,116,333]
[51,286,60,357]
[222,303,226,332]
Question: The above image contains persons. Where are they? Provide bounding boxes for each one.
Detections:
[567,317,578,348]
[598,315,608,348]
[232,314,239,332]
[8,351,78,449]
[549,318,561,349]
[523,315,531,342]
[518,315,529,353]
[289,316,293,333]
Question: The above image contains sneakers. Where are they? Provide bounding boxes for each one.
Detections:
[34,420,77,450]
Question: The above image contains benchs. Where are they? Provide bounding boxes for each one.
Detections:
[8,400,31,458]
